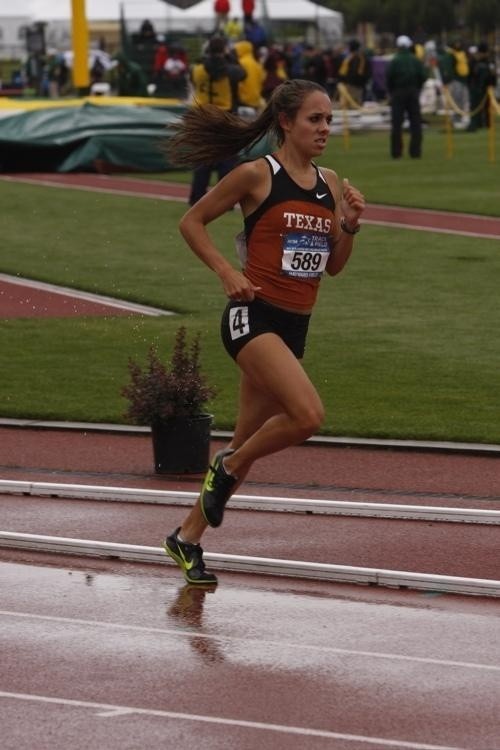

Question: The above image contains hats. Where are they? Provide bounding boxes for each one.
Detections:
[396,35,411,49]
[140,20,154,32]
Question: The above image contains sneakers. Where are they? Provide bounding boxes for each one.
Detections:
[160,526,219,584]
[200,447,238,528]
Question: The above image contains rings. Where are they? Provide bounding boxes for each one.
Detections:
[236,298,242,302]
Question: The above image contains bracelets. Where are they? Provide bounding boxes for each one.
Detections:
[340,218,362,234]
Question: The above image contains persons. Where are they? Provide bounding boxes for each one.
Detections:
[165,583,224,663]
[178,30,249,215]
[25,18,190,97]
[383,31,427,162]
[424,40,498,133]
[228,17,388,110]
[159,77,368,585]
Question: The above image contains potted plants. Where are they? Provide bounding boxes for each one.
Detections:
[115,323,221,477]
